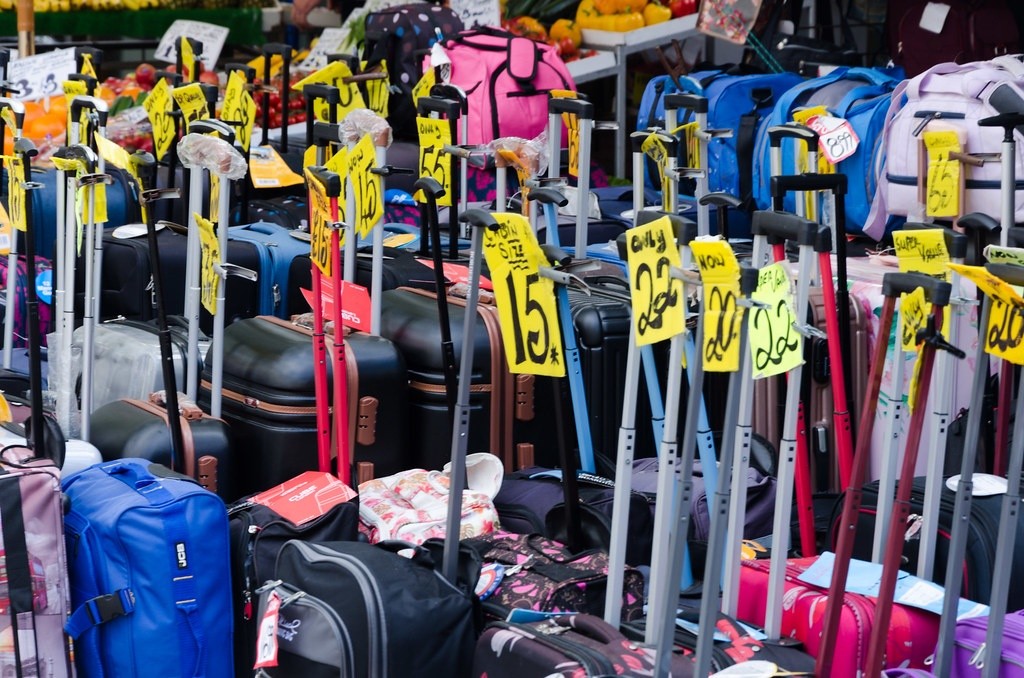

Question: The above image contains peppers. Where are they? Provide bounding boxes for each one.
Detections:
[108,92,148,116]
[500,0,697,64]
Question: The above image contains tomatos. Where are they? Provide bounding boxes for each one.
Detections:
[251,71,315,128]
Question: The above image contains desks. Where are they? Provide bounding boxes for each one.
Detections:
[570,29,716,180]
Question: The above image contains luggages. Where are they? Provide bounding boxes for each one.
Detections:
[0,46,1024,678]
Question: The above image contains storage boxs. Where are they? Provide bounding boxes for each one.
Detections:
[582,12,699,45]
[564,48,616,77]
[297,27,352,76]
[243,0,283,32]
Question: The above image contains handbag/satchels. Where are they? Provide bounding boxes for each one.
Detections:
[352,4,465,139]
[886,0,1020,78]
[636,62,805,211]
[751,66,910,242]
[422,25,578,148]
[863,55,1024,242]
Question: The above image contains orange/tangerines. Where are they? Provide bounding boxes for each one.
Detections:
[100,86,144,104]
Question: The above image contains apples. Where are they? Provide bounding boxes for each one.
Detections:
[105,63,218,91]
